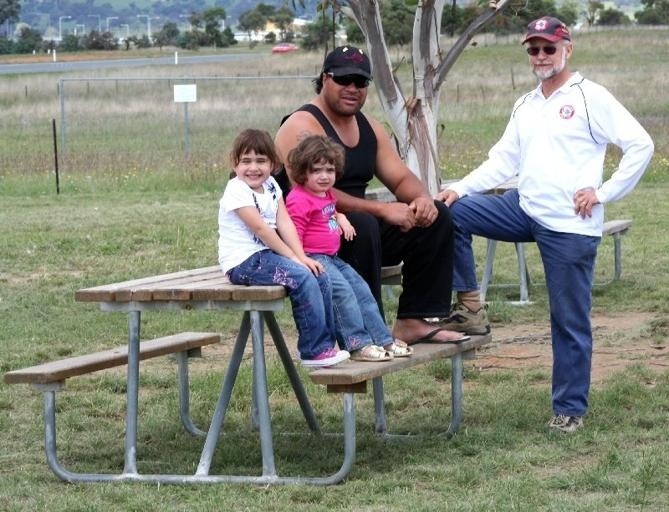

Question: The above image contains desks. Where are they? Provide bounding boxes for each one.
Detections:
[73,262,406,484]
[442,175,538,306]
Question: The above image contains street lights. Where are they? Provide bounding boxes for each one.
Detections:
[58,7,232,36]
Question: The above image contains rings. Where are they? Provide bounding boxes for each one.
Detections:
[581,201,587,205]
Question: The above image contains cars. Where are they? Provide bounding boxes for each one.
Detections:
[270,40,298,54]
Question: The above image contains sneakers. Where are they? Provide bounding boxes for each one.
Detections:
[300,348,351,369]
[548,413,584,433]
[431,302,491,337]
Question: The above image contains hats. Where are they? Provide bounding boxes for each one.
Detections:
[324,46,372,81]
[521,17,571,46]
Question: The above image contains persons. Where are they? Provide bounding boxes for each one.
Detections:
[268,45,470,342]
[430,15,655,431]
[216,128,352,368]
[285,135,416,361]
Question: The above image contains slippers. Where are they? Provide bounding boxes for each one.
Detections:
[407,327,472,347]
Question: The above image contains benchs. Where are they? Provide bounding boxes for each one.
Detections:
[310,318,493,485]
[601,218,633,290]
[5,331,222,485]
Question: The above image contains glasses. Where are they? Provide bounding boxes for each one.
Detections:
[525,46,556,57]
[330,75,370,90]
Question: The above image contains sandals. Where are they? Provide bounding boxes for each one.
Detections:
[351,343,394,362]
[385,339,414,358]
